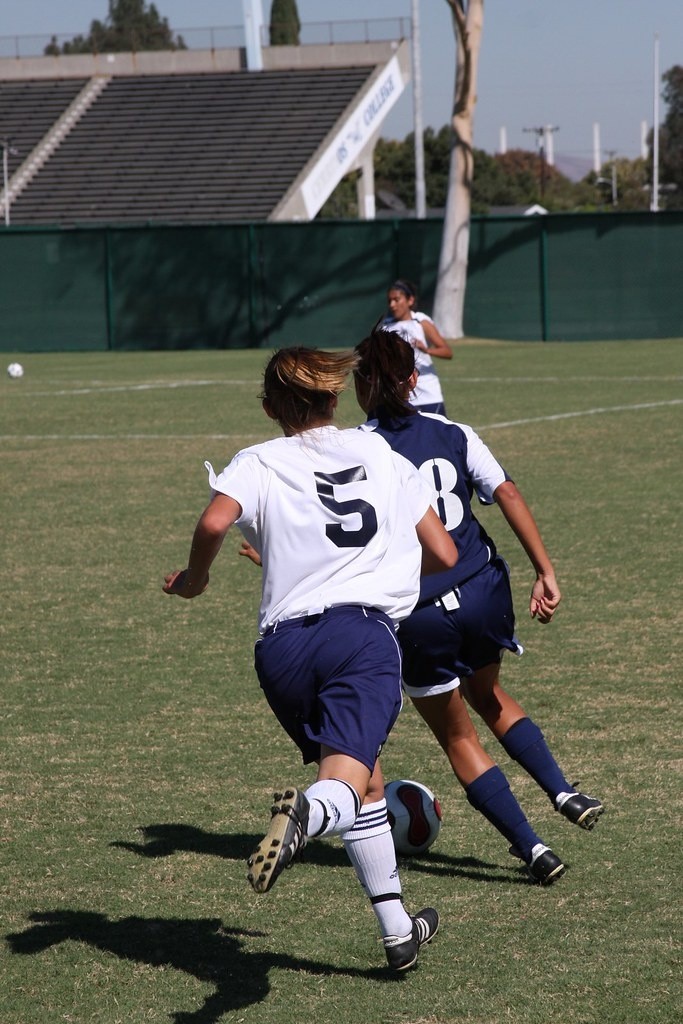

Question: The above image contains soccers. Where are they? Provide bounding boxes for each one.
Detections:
[381,778,444,857]
[7,363,24,379]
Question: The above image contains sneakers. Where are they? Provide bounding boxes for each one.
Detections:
[555,781,605,831]
[247,786,310,894]
[509,844,565,886]
[383,907,441,975]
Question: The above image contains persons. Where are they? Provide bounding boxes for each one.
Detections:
[369,281,454,420]
[163,346,458,972]
[238,327,603,884]
